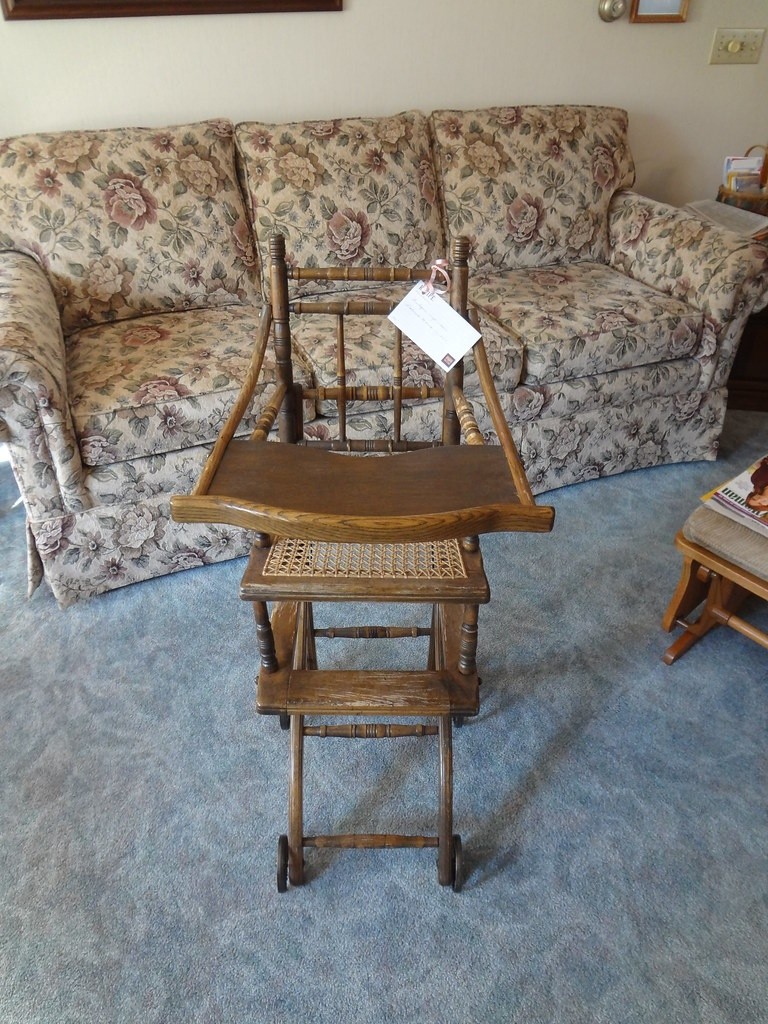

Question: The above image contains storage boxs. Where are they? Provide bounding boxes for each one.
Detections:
[717,143,767,217]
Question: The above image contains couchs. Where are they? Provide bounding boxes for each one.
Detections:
[2,103,767,609]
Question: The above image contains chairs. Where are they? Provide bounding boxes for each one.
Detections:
[664,451,768,667]
[170,226,557,892]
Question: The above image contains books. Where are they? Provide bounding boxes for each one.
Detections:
[721,155,763,194]
[700,471,739,503]
[704,452,768,546]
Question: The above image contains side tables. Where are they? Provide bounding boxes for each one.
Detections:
[727,308,768,412]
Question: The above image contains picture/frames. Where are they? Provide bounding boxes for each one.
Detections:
[629,0,689,24]
[1,0,342,20]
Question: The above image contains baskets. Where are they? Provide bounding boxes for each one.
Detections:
[715,146,768,217]
